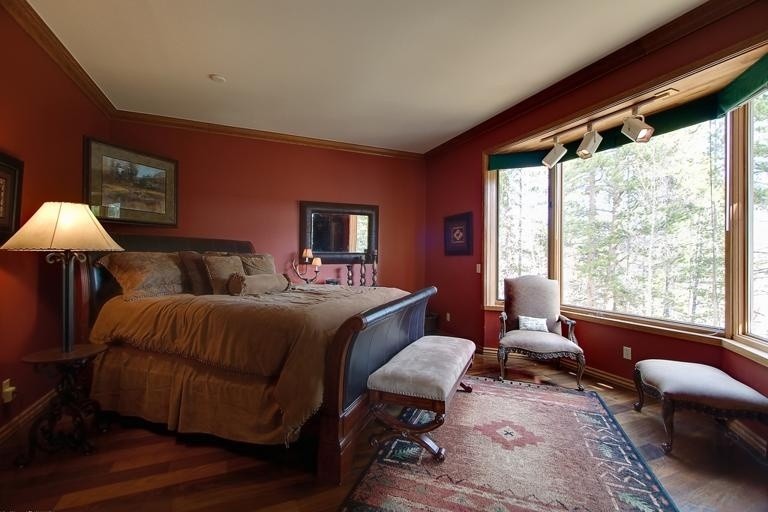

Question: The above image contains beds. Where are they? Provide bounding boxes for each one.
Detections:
[75,230,440,485]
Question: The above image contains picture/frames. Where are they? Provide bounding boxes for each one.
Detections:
[442,210,474,257]
[79,135,177,230]
[1,153,24,246]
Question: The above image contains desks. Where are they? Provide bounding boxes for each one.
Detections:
[23,343,111,452]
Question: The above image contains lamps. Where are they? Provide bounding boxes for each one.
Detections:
[538,102,654,170]
[291,248,322,284]
[1,202,126,354]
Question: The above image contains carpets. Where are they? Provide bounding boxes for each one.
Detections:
[336,372,682,511]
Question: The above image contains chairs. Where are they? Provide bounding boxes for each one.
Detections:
[495,273,586,391]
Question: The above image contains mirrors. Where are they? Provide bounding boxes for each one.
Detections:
[296,200,380,264]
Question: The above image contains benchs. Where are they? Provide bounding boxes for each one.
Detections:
[366,333,479,461]
[634,358,768,455]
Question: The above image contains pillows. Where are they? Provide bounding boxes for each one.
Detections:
[206,251,273,275]
[240,255,276,274]
[93,250,194,302]
[517,315,550,333]
[201,254,248,296]
[227,272,292,296]
[179,249,212,296]
